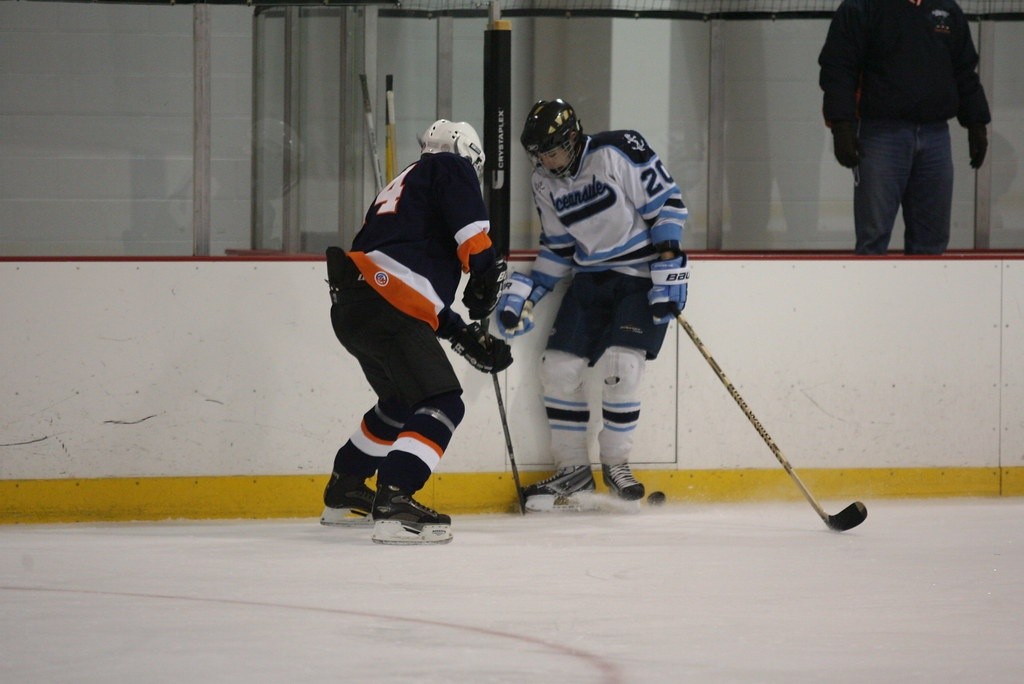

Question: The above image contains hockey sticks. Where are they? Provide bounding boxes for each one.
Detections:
[481,316,527,513]
[670,303,868,532]
[359,72,386,196]
[380,72,396,184]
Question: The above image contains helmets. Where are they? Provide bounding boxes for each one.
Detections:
[521,98,582,181]
[420,119,486,184]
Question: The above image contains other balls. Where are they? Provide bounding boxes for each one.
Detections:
[647,491,666,507]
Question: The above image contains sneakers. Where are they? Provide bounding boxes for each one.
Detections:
[602,462,646,513]
[371,470,453,545]
[523,465,596,513]
[321,453,377,528]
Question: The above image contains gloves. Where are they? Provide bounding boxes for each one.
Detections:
[461,253,507,320]
[968,127,988,169]
[451,322,514,373]
[496,271,549,338]
[833,128,864,169]
[648,251,690,325]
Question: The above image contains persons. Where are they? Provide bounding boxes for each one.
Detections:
[157,119,304,257]
[495,99,690,513]
[817,0,992,255]
[319,119,513,545]
